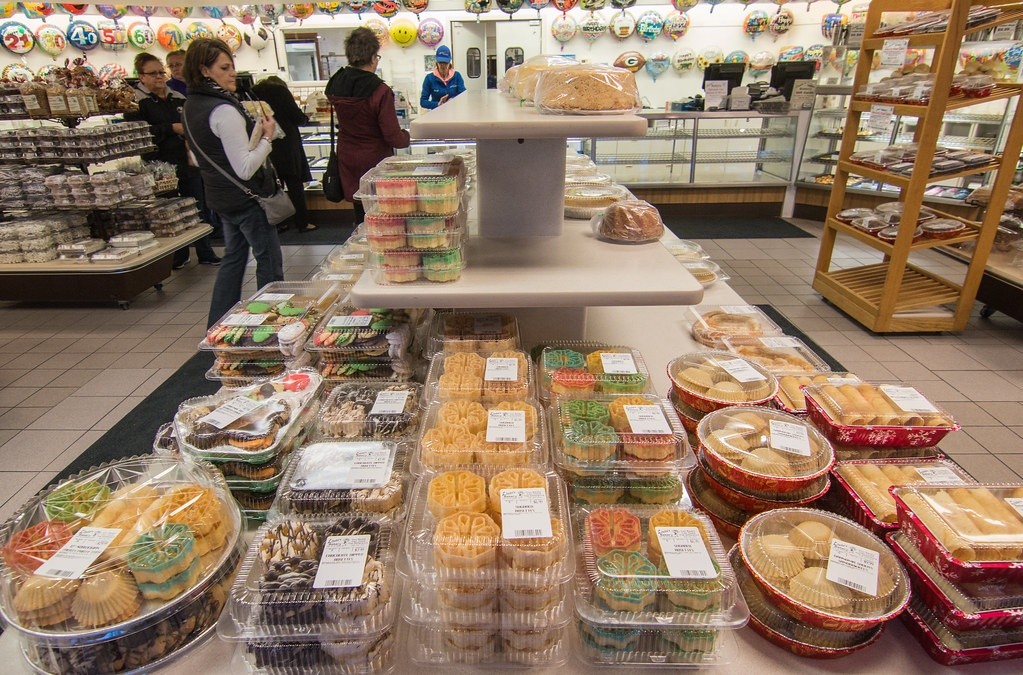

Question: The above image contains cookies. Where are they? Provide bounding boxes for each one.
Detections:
[208,303,414,386]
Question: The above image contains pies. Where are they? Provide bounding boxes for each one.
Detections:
[693,310,763,346]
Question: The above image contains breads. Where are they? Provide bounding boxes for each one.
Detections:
[507,64,637,111]
[746,517,894,647]
[565,159,663,240]
[706,412,820,522]
[9,572,143,627]
[678,359,770,401]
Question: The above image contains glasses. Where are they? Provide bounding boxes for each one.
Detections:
[166,63,182,68]
[141,71,167,77]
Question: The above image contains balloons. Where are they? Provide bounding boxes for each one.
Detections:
[0,0,444,84]
[463,0,1023,84]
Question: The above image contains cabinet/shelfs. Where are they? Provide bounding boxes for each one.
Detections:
[794,45,1023,221]
[812,0,1023,337]
[0,89,1022,675]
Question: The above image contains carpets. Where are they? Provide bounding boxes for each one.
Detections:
[193,217,360,246]
[34,350,223,496]
[754,304,848,373]
[661,215,818,240]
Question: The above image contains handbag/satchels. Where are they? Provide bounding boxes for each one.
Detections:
[255,189,296,226]
[321,152,343,202]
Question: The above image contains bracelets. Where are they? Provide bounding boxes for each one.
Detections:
[260,136,273,143]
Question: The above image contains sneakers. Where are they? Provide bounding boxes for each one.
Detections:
[299,223,319,233]
[172,249,191,268]
[197,248,223,265]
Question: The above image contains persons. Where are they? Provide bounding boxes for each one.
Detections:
[252,76,320,233]
[505,56,515,71]
[181,37,283,337]
[324,27,410,230]
[420,45,466,109]
[121,49,222,269]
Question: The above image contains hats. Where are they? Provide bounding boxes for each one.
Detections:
[435,45,452,63]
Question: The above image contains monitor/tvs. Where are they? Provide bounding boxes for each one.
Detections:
[774,61,816,102]
[703,63,746,96]
